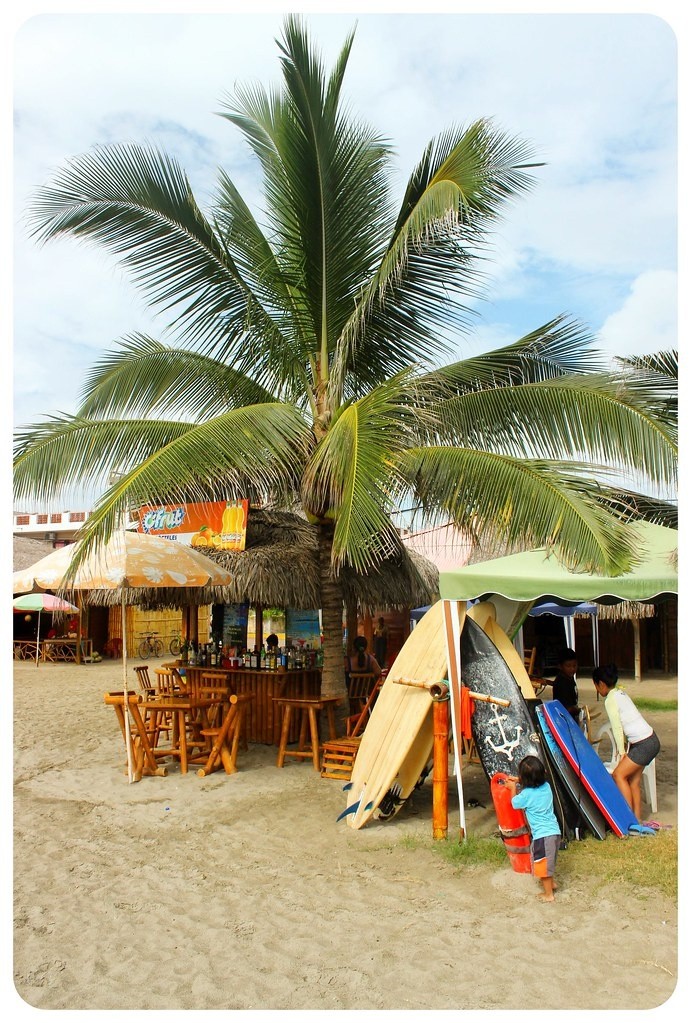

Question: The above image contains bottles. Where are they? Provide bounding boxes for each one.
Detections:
[181,639,323,673]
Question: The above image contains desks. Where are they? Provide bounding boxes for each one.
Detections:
[136,696,219,774]
[271,695,344,773]
[44,638,93,663]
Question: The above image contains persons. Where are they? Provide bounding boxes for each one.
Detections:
[551,648,582,724]
[317,636,382,696]
[591,661,661,822]
[373,617,390,665]
[265,634,278,648]
[502,755,561,902]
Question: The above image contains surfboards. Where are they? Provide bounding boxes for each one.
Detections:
[337,594,641,874]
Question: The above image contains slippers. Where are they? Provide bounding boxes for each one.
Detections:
[641,827,655,834]
[629,824,642,835]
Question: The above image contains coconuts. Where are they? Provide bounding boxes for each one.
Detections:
[25,615,31,622]
[91,651,102,663]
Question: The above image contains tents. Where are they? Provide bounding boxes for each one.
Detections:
[410,599,598,680]
[438,519,678,841]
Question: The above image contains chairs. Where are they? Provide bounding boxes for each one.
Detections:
[348,672,375,735]
[106,690,165,766]
[187,686,231,755]
[133,665,173,723]
[104,695,168,776]
[202,672,249,751]
[155,669,194,741]
[524,647,537,676]
[593,721,658,813]
[197,691,258,777]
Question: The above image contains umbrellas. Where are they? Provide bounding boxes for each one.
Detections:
[13,529,235,783]
[13,593,79,663]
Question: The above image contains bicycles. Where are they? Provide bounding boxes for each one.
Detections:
[138,631,164,660]
[169,630,182,656]
